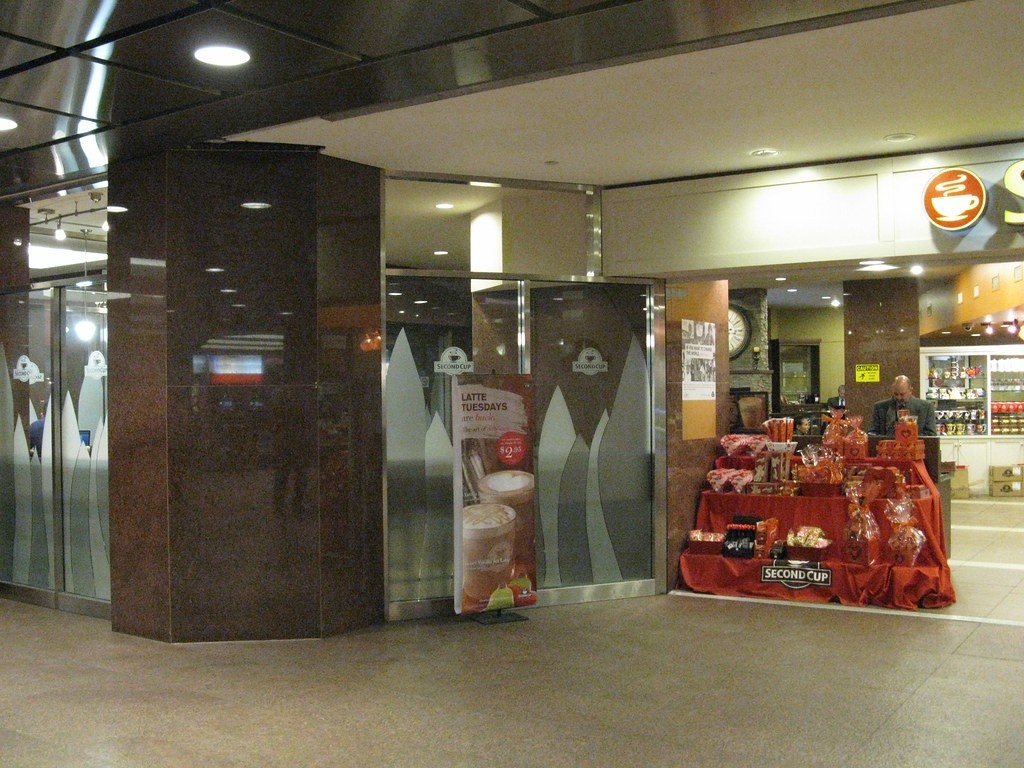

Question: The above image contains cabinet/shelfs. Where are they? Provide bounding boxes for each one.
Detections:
[919,344,1023,439]
[939,439,990,494]
[989,439,1024,464]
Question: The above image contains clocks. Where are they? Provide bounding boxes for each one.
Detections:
[727,303,751,362]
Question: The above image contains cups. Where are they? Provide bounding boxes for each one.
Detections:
[933,410,987,435]
[462,470,537,613]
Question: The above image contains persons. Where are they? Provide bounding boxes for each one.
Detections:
[870,375,937,436]
[794,415,811,455]
[820,385,846,435]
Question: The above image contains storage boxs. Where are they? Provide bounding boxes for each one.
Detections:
[990,482,1024,498]
[688,421,926,568]
[949,470,984,499]
[989,464,1024,483]
